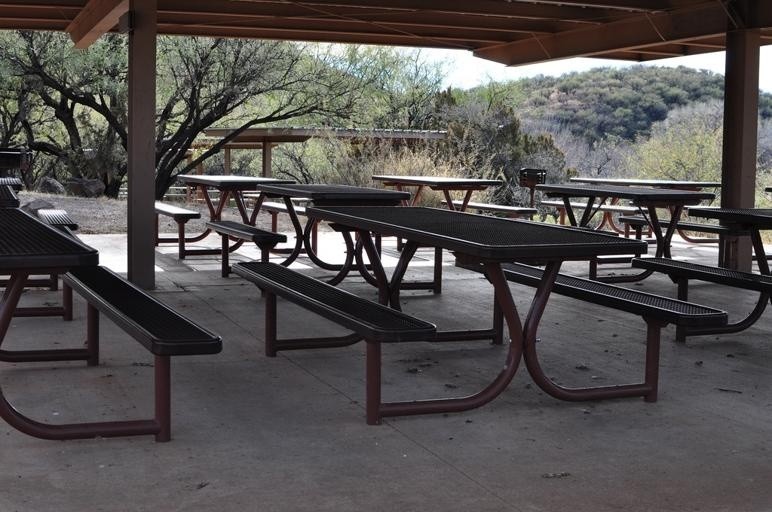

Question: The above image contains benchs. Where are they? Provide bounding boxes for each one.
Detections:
[153,175,770,425]
[1,175,222,442]
